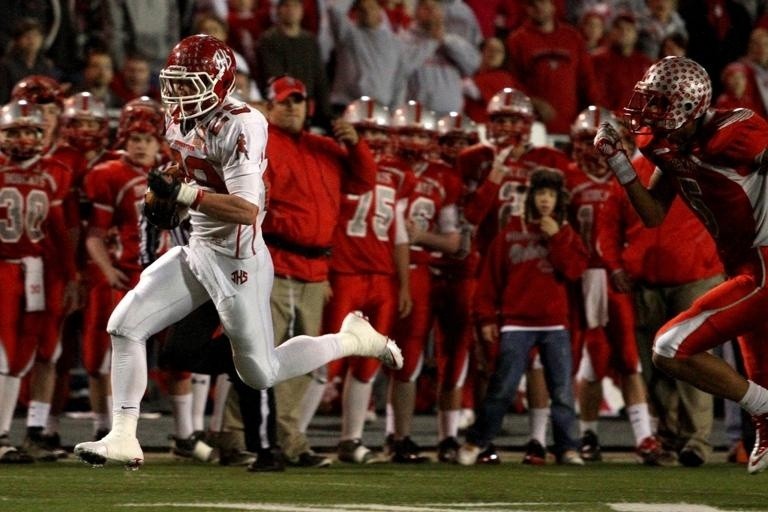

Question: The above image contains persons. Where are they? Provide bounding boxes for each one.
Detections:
[2,2,768,476]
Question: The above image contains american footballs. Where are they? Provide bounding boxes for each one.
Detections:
[143,172,180,224]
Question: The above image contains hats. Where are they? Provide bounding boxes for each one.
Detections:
[519,166,565,226]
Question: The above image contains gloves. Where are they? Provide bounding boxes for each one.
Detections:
[143,202,180,230]
[147,165,181,202]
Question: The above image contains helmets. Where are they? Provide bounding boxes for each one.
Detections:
[621,57,712,171]
[0,33,616,168]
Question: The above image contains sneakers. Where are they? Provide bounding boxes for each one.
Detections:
[172,429,709,469]
[747,413,768,472]
[22,426,68,460]
[340,310,404,371]
[74,428,144,466]
[2,433,20,463]
